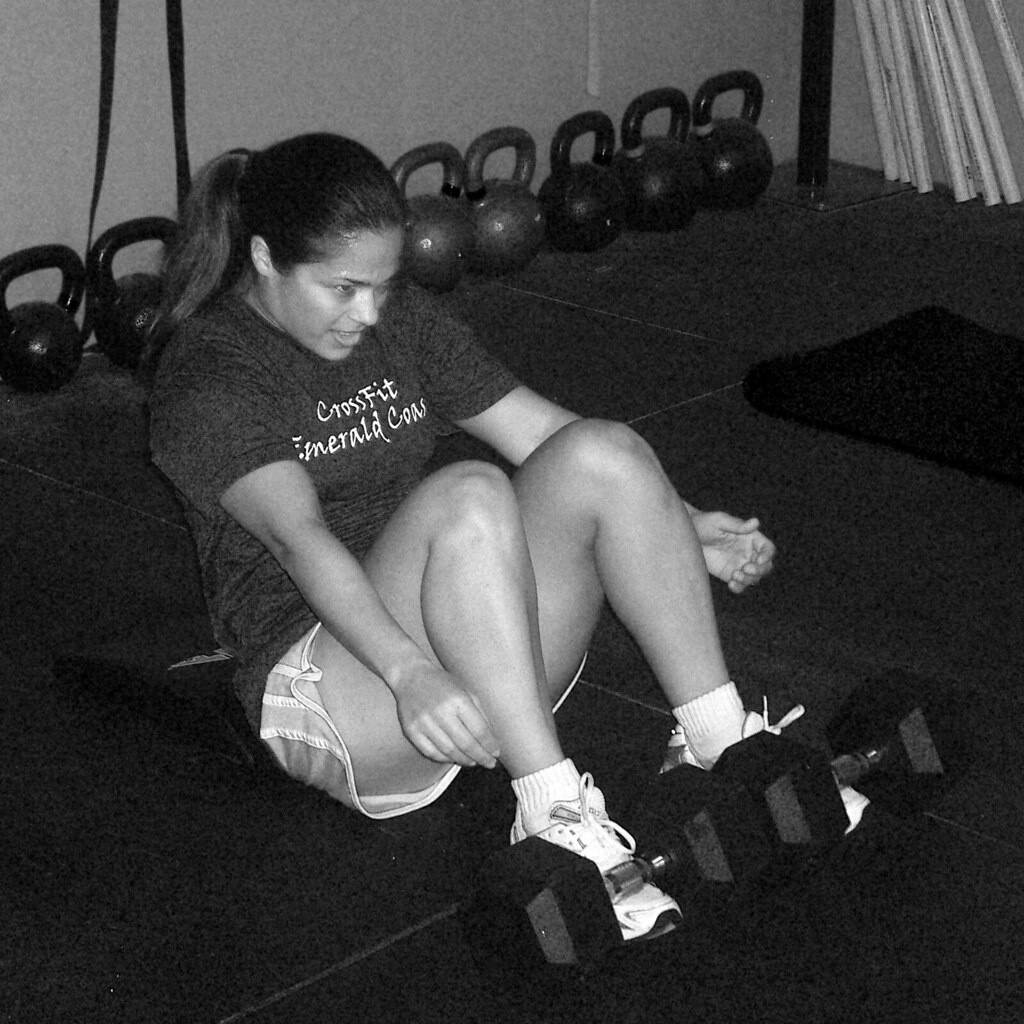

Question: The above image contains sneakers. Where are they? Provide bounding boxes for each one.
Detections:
[659,710,872,837]
[511,785,683,942]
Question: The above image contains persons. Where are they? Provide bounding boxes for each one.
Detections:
[142,129,869,945]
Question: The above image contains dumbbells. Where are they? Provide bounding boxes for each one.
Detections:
[452,762,772,998]
[713,668,973,883]
